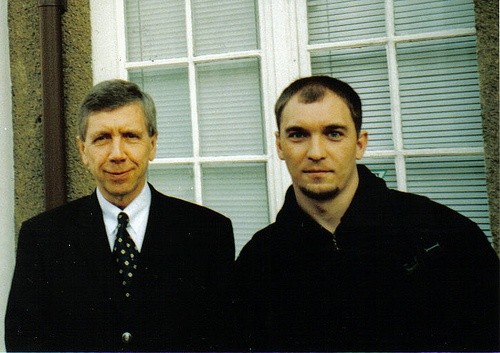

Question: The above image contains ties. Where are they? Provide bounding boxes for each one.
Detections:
[113,212,140,299]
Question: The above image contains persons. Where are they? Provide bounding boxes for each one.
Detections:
[4,77,236,353]
[214,75,500,353]
